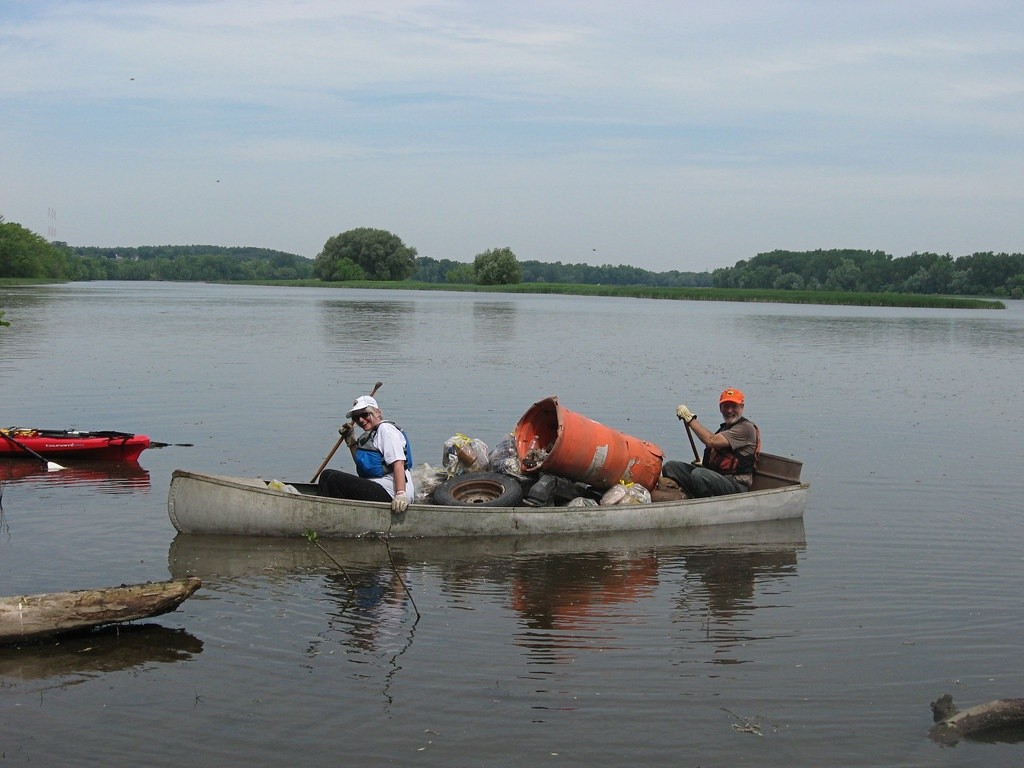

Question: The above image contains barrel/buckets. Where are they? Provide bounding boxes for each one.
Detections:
[515,396,662,491]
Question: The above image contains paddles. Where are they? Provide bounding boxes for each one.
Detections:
[682,418,701,462]
[41,429,193,449]
[0,430,66,471]
[310,382,383,485]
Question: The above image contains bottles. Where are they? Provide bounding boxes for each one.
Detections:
[470,442,489,466]
[525,435,539,457]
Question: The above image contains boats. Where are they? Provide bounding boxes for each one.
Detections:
[168,466,811,539]
[0,427,151,463]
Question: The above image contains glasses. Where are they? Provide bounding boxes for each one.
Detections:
[350,412,373,421]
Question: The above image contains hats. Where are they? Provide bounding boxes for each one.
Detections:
[718,388,747,407]
[346,396,379,419]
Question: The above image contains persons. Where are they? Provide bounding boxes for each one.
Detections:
[317,396,415,514]
[661,388,760,499]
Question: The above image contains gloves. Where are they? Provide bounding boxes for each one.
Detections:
[340,422,358,447]
[676,404,698,425]
[392,491,409,514]
[691,458,711,467]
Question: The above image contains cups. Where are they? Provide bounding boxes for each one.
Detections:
[456,446,477,466]
